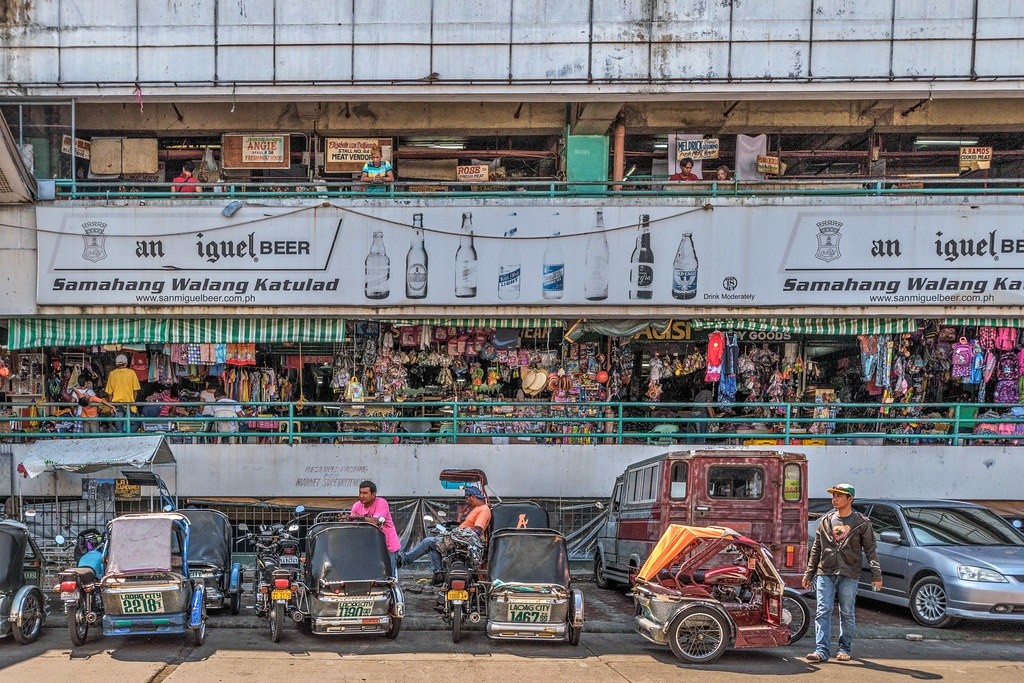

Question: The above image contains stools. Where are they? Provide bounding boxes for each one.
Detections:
[319,437,337,444]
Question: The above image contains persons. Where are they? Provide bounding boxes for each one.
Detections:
[199,374,244,444]
[717,166,733,180]
[338,481,401,576]
[361,146,395,191]
[70,374,116,438]
[670,157,699,180]
[802,484,882,661]
[171,160,203,198]
[105,354,141,433]
[395,486,491,585]
[691,384,712,444]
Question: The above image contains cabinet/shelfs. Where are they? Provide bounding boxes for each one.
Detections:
[341,405,394,443]
[6,353,49,431]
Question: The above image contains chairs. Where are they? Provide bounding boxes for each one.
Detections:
[214,410,242,444]
[647,424,679,445]
[278,421,302,444]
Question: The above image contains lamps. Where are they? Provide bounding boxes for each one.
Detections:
[623,164,638,181]
[407,143,468,149]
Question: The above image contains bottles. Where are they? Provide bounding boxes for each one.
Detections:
[364,230,390,299]
[628,215,655,300]
[405,213,428,299]
[541,212,565,300]
[497,211,521,301]
[453,213,477,299]
[671,231,700,300]
[583,209,610,301]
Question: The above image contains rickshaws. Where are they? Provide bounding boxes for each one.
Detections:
[0,519,51,644]
[174,507,250,613]
[232,505,423,644]
[424,499,552,535]
[428,524,587,643]
[52,512,211,646]
[626,524,813,665]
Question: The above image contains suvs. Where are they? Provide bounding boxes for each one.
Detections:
[592,449,813,596]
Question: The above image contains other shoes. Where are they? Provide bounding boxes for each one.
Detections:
[837,651,851,661]
[430,572,444,586]
[806,652,828,663]
[397,557,402,569]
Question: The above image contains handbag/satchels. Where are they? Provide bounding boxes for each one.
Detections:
[329,343,365,410]
[890,318,1024,447]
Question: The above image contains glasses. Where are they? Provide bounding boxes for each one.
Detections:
[833,485,854,496]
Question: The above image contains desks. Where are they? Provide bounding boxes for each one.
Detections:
[651,409,691,445]
[724,429,771,445]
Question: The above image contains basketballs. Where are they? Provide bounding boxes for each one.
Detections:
[596,371,609,383]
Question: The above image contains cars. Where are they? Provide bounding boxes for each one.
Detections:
[814,499,1023,629]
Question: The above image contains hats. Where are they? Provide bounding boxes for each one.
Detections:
[827,483,855,498]
[465,486,486,499]
[116,355,127,365]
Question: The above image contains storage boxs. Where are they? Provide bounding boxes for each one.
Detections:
[812,437,856,445]
[847,433,885,446]
[173,421,209,431]
[744,439,778,445]
[803,439,827,445]
[777,439,803,445]
[143,421,173,431]
